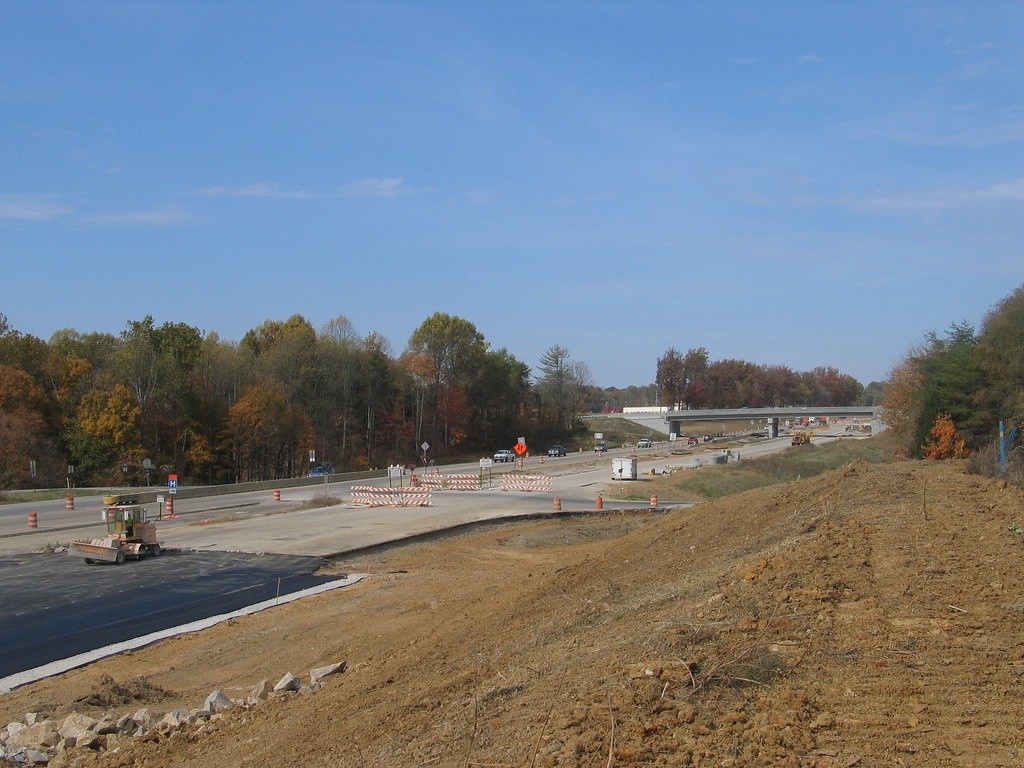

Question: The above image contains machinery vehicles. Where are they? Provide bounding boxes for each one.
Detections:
[791,431,810,446]
[67,505,165,564]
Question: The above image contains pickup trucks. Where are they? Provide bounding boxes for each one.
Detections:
[493,450,515,463]
[703,434,714,443]
[688,436,698,444]
[594,443,607,452]
[548,445,567,457]
[637,439,652,448]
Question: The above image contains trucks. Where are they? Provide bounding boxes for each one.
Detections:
[795,416,814,426]
[818,416,828,426]
